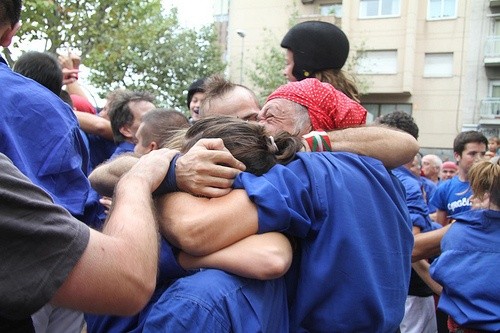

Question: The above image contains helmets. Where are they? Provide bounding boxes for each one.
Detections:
[186,77,207,109]
[280,21,349,82]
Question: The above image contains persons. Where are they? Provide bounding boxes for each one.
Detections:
[0,0,500,333]
[280,21,366,105]
